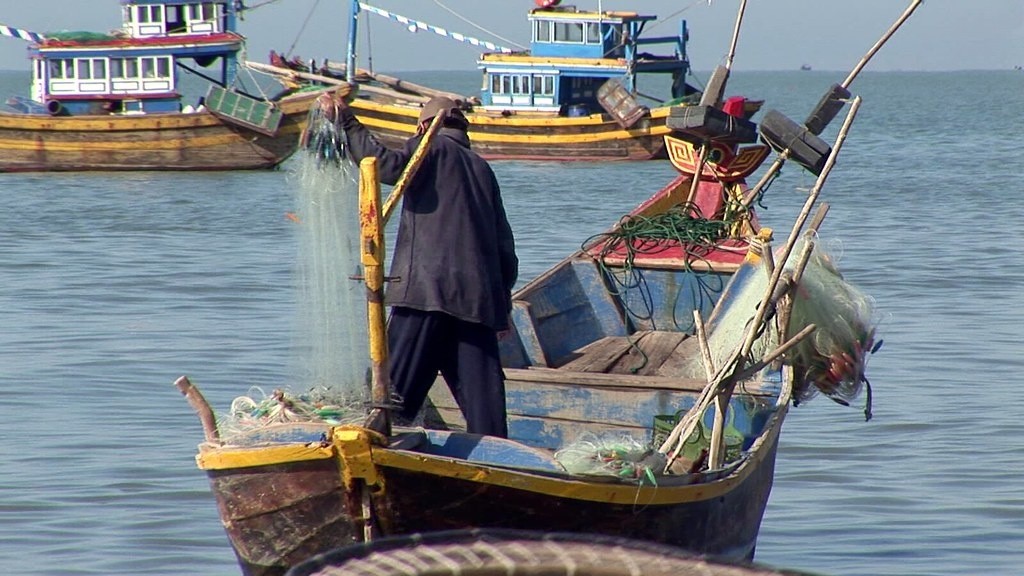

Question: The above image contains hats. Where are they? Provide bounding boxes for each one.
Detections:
[417,95,468,124]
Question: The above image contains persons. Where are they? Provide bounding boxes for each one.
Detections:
[318,94,520,440]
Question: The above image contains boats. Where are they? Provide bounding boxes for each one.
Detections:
[0,1,362,177]
[174,0,930,576]
[239,1,768,166]
